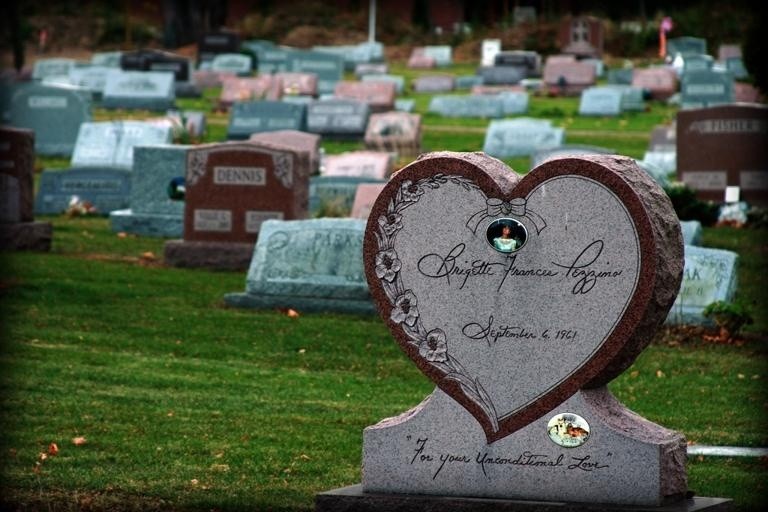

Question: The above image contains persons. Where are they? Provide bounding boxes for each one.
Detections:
[493,222,518,251]
